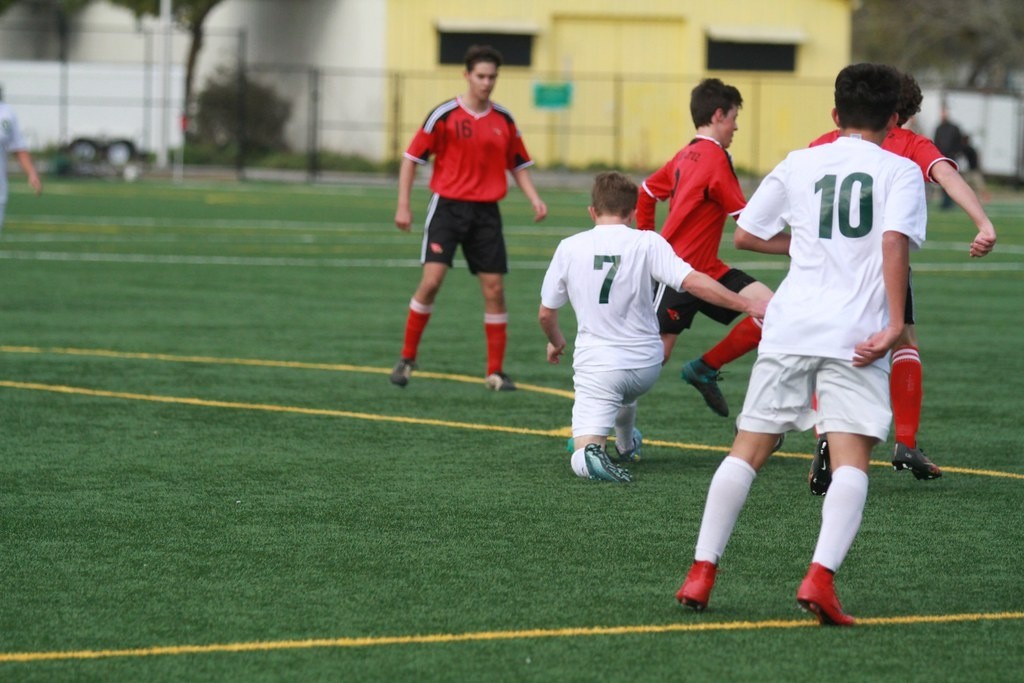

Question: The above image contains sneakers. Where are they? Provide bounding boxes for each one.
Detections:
[584,443,633,483]
[808,433,832,496]
[389,357,415,386]
[486,371,516,390]
[675,560,717,612]
[623,427,643,461]
[681,358,729,416]
[892,441,943,480]
[797,563,853,626]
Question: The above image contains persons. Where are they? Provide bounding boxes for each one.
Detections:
[676,63,927,626]
[809,72,991,499]
[566,80,776,456]
[0,84,43,237]
[538,172,770,485]
[390,46,548,393]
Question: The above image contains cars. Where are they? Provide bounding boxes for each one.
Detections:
[907,77,1024,190]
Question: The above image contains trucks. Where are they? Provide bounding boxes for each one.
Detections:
[0,57,189,176]
